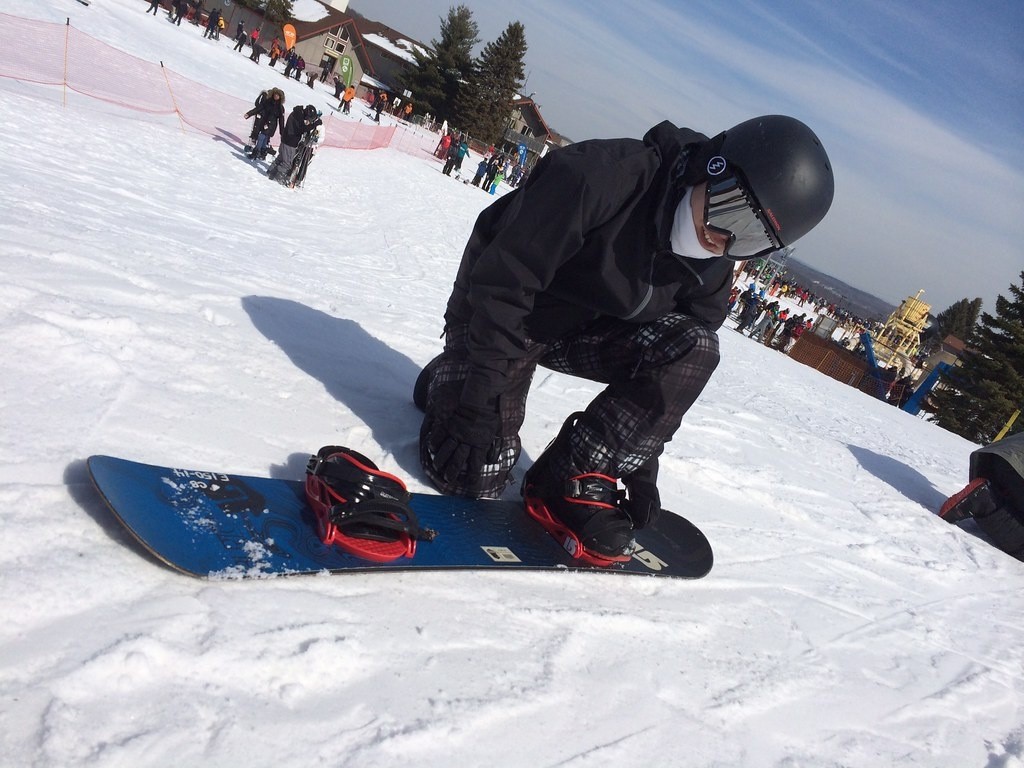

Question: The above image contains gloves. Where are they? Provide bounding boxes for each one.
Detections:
[431,402,503,481]
[315,119,322,125]
[620,463,662,530]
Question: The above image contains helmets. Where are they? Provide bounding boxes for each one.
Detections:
[306,105,316,119]
[686,114,834,247]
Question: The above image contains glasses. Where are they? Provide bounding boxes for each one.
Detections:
[704,163,780,261]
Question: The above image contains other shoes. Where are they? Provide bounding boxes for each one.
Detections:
[413,353,449,412]
[525,453,633,557]
[247,154,265,161]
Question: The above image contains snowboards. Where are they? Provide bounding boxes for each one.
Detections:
[86,446,717,583]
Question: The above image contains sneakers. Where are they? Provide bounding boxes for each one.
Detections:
[938,477,1003,523]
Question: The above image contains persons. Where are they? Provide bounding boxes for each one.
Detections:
[936,433,1024,563]
[143,0,916,411]
[413,113,836,566]
